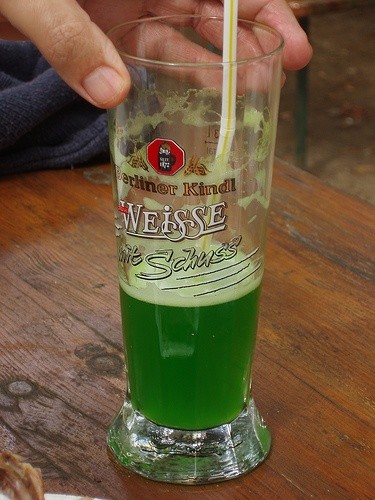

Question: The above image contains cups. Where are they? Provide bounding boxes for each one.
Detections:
[105,15,283,485]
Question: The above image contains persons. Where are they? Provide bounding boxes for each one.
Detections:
[0,0,315,169]
